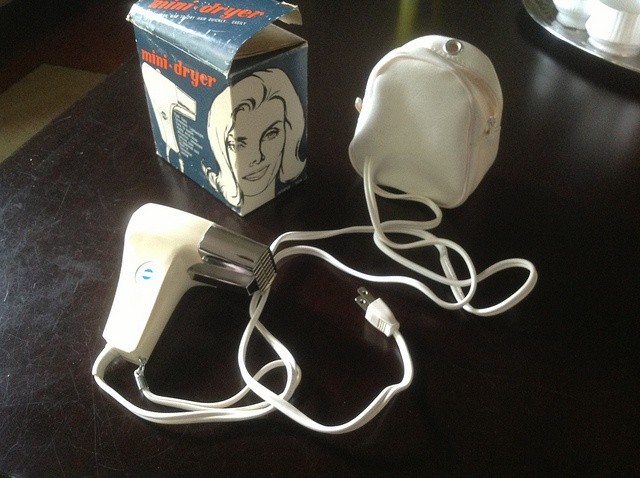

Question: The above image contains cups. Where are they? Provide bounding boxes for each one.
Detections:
[552,0,589,31]
[584,1,640,56]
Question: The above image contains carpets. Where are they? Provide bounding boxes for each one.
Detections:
[0,63,109,165]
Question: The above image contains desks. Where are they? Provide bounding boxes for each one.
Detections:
[0,1,640,476]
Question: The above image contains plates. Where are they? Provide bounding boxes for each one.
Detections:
[523,0,640,74]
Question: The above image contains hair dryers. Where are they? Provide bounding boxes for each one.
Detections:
[93,203,277,377]
